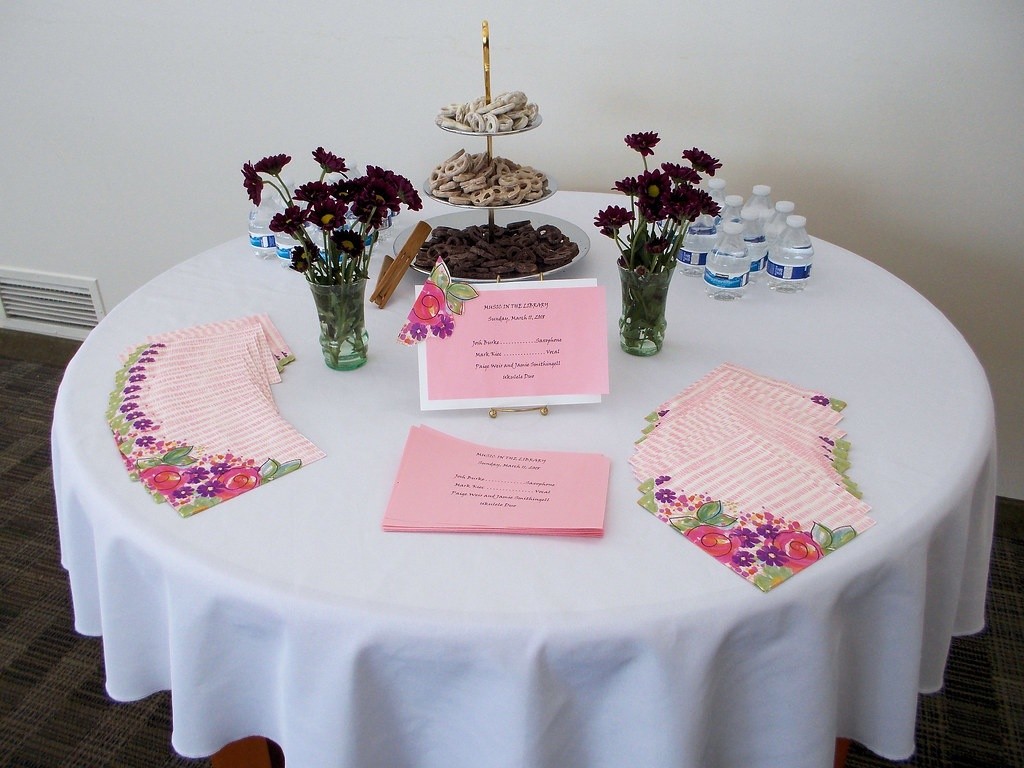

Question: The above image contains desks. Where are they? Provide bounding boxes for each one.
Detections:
[52,191,995,768]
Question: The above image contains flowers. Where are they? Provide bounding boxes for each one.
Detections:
[592,132,719,273]
[240,147,421,283]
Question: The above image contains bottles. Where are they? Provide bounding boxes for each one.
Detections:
[677,169,812,302]
[249,174,398,276]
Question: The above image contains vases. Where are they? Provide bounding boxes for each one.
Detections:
[304,275,368,372]
[616,256,676,357]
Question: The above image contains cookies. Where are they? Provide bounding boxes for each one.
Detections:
[428,149,549,205]
[434,90,541,133]
[414,219,579,278]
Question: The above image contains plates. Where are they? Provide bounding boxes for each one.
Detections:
[424,175,556,208]
[434,114,542,136]
[393,209,590,283]
